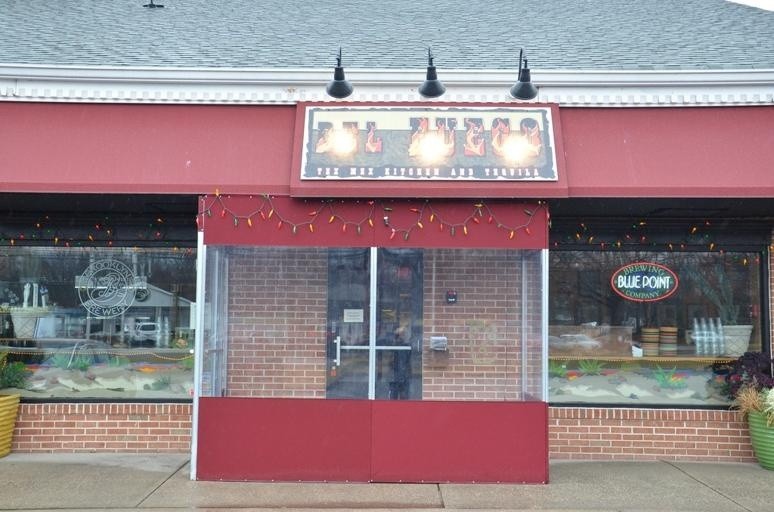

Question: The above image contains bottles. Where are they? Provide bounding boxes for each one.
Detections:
[693,316,725,356]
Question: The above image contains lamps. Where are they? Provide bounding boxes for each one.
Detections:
[325,45,353,99]
[509,46,538,100]
[418,45,446,98]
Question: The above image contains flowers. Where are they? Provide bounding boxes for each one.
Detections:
[711,351,774,428]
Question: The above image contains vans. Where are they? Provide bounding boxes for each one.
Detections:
[130,322,159,347]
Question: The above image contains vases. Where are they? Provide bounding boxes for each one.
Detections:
[747,411,774,471]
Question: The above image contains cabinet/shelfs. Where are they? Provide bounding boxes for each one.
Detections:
[0,307,195,359]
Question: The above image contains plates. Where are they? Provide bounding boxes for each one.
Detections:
[640,326,677,357]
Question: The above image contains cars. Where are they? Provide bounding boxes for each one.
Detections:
[560,334,601,352]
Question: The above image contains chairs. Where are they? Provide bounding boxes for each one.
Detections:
[10,314,56,339]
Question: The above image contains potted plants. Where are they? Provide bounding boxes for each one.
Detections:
[0,348,33,458]
[682,263,753,358]
